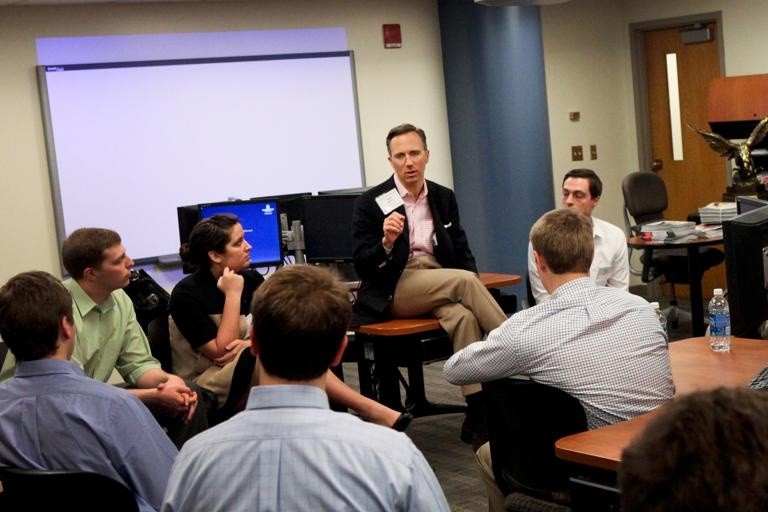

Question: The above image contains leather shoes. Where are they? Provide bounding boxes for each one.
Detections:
[392,412,414,430]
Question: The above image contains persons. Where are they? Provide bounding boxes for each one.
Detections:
[168,214,413,432]
[619,386,768,512]
[160,264,451,512]
[443,208,676,512]
[0,271,179,512]
[351,123,530,453]
[528,168,630,305]
[0,228,208,448]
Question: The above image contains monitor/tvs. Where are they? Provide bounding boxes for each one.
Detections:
[306,196,362,263]
[199,201,284,267]
[721,205,768,339]
[178,205,201,273]
[250,193,313,255]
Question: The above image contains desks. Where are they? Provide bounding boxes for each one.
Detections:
[139,255,362,374]
[556,337,767,503]
[477,274,521,288]
[626,222,724,337]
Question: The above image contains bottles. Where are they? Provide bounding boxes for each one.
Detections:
[708,288,731,352]
[650,302,668,344]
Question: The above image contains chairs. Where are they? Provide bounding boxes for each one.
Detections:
[0,465,139,512]
[622,171,724,342]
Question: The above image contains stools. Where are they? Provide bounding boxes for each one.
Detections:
[359,320,467,419]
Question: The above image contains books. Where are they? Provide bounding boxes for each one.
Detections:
[696,224,724,238]
[640,220,696,244]
[698,202,738,224]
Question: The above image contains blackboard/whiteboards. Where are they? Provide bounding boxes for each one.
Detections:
[37,50,367,277]
